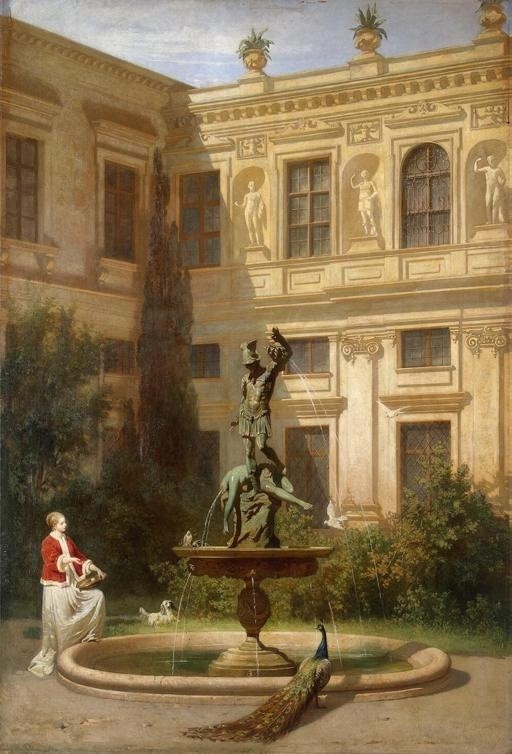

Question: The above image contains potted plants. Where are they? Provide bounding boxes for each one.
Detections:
[235,25,275,73]
[475,0,507,28]
[350,2,386,55]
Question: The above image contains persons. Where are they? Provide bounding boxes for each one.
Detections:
[231,327,293,500]
[234,180,265,247]
[350,170,378,235]
[473,155,508,224]
[28,511,107,677]
